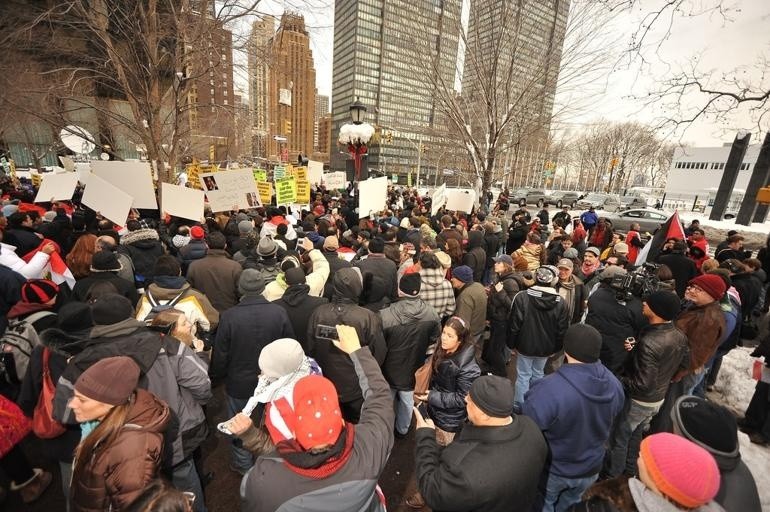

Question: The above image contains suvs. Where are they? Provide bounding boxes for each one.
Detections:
[508,188,551,207]
[549,190,578,208]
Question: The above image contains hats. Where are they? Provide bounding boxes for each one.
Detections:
[74,355,140,406]
[89,250,123,272]
[687,274,726,301]
[646,289,680,322]
[280,250,300,272]
[91,294,134,327]
[56,302,93,332]
[237,268,265,296]
[557,259,573,270]
[563,247,578,259]
[324,236,339,251]
[255,236,279,256]
[21,278,59,304]
[670,394,741,472]
[302,221,315,231]
[613,243,629,254]
[42,211,56,222]
[189,226,204,240]
[562,322,603,363]
[597,264,628,281]
[358,230,370,238]
[491,254,512,265]
[536,264,559,284]
[236,212,248,224]
[453,265,474,283]
[399,273,421,295]
[469,374,514,418]
[285,267,306,284]
[238,220,254,234]
[639,431,721,509]
[584,247,601,256]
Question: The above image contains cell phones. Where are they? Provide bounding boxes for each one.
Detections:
[313,324,339,342]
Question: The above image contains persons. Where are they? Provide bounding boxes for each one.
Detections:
[0,393,53,503]
[689,247,709,269]
[506,265,570,414]
[417,253,457,324]
[657,240,700,299]
[604,255,636,271]
[656,237,680,255]
[407,315,482,509]
[135,256,221,348]
[600,243,630,264]
[376,273,443,438]
[599,291,688,481]
[688,230,709,254]
[0,279,59,415]
[651,274,725,432]
[208,268,297,447]
[269,269,328,332]
[556,258,587,323]
[688,219,699,230]
[701,259,734,286]
[121,482,193,512]
[53,293,213,512]
[73,250,141,311]
[668,395,762,511]
[308,267,388,425]
[692,270,737,397]
[714,234,751,262]
[0,166,390,274]
[727,230,737,237]
[412,375,548,512]
[389,185,613,261]
[225,337,318,457]
[589,263,658,377]
[756,233,770,312]
[240,324,395,511]
[742,260,765,284]
[569,431,728,512]
[576,247,605,282]
[451,264,487,372]
[719,258,762,319]
[612,233,624,244]
[67,356,171,512]
[521,323,625,512]
[626,222,645,264]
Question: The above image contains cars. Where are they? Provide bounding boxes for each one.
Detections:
[600,208,686,235]
[576,193,621,213]
[610,195,647,211]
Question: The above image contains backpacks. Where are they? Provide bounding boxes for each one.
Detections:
[145,289,189,328]
[1,311,57,385]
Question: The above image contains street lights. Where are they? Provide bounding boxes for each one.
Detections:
[349,95,366,181]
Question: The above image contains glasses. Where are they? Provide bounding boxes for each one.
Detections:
[605,260,616,265]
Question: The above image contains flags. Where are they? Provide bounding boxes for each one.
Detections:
[630,210,686,267]
[21,239,76,290]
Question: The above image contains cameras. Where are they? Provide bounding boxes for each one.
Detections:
[607,261,660,305]
[296,237,305,245]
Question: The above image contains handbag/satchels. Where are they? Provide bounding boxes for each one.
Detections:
[413,353,432,405]
